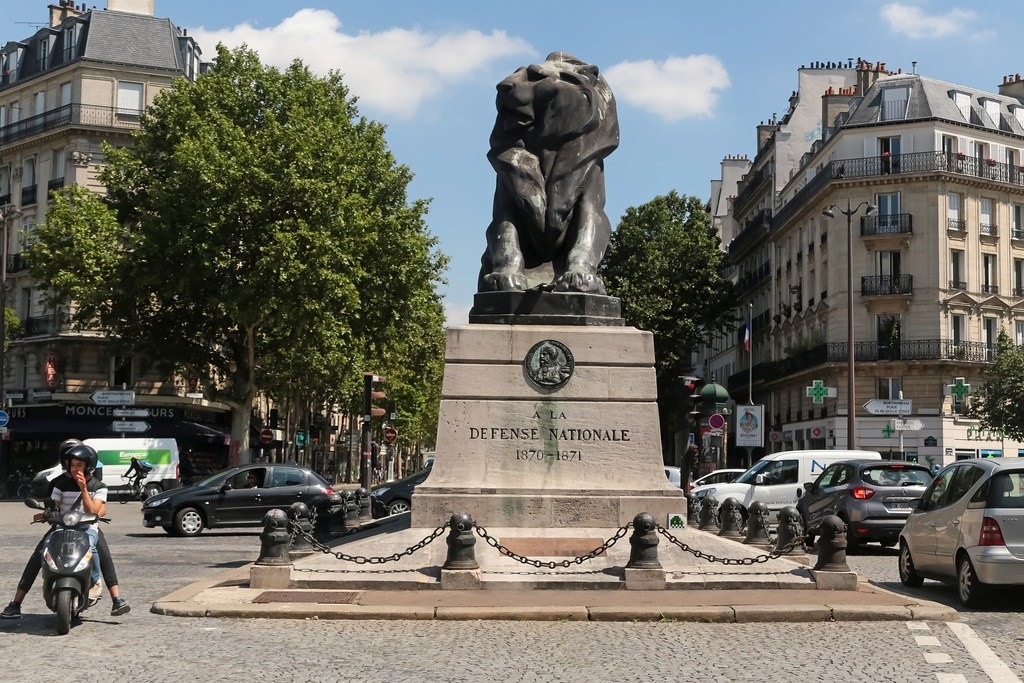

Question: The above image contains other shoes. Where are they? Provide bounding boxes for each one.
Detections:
[1,602,21,618]
[111,599,130,616]
[133,492,142,501]
[87,579,103,600]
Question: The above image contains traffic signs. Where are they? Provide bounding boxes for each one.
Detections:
[113,421,151,433]
[892,418,926,431]
[863,398,913,415]
[115,409,152,418]
[87,389,135,406]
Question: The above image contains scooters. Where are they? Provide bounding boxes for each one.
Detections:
[24,497,112,635]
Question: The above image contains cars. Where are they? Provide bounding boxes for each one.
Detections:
[689,467,746,488]
[371,463,433,518]
[139,462,343,538]
[899,455,1023,606]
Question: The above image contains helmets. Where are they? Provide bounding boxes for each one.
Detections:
[63,445,98,476]
[59,439,83,470]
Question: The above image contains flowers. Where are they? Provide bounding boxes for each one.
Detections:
[956,153,966,157]
[985,159,996,164]
[881,152,890,156]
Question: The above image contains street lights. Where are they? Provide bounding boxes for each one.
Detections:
[821,197,879,454]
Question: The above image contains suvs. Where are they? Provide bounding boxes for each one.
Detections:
[796,458,948,550]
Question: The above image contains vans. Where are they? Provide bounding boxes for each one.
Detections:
[684,448,883,533]
[27,437,183,502]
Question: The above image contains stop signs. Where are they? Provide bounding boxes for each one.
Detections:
[259,429,274,444]
[385,428,397,441]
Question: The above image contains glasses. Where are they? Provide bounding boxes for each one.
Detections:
[247,476,256,480]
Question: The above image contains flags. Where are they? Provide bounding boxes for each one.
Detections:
[744,319,750,352]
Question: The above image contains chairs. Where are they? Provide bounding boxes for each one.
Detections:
[1004,475,1014,497]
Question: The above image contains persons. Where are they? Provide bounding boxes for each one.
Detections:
[124,457,147,496]
[702,432,716,462]
[34,445,108,599]
[0,439,130,617]
[931,464,940,476]
[247,471,259,489]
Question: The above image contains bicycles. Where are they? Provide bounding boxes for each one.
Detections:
[116,474,155,504]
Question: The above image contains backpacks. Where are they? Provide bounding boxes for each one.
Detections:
[134,458,152,471]
